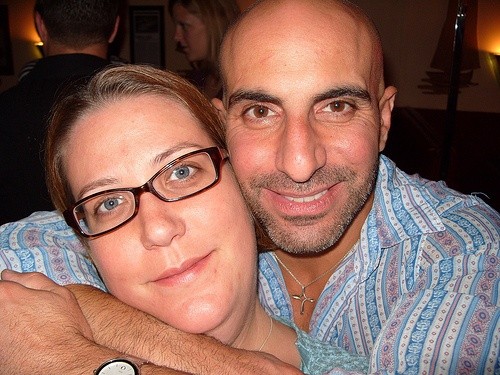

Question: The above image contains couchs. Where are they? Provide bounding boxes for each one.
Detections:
[382,107,500,214]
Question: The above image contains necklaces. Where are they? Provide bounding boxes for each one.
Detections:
[258,317,273,352]
[272,243,355,314]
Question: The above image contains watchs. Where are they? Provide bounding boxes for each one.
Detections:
[93,358,139,375]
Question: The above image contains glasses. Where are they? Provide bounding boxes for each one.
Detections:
[62,145,230,240]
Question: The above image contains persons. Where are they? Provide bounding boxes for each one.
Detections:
[0,63,369,375]
[0,0,128,227]
[168,0,247,102]
[0,0,500,375]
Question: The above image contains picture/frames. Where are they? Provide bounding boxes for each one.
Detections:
[0,4,14,76]
[128,5,165,71]
